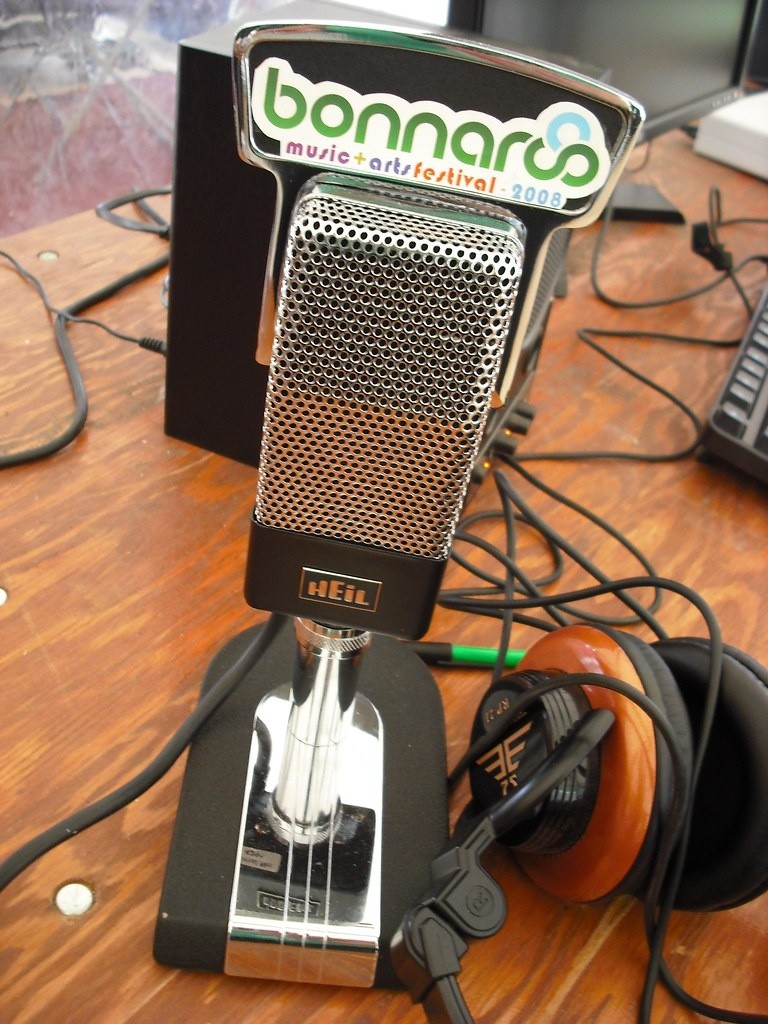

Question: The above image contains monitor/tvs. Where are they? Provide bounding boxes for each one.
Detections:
[445,0,763,227]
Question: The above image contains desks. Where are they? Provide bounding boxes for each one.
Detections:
[0,111,767,1024]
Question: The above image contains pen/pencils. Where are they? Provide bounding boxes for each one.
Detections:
[406,639,526,666]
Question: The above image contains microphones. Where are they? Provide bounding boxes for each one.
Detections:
[242,169,533,847]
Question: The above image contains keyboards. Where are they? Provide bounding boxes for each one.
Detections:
[703,284,768,485]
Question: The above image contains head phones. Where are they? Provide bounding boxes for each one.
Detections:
[386,621,768,1024]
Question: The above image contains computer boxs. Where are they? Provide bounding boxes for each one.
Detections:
[160,0,575,506]
